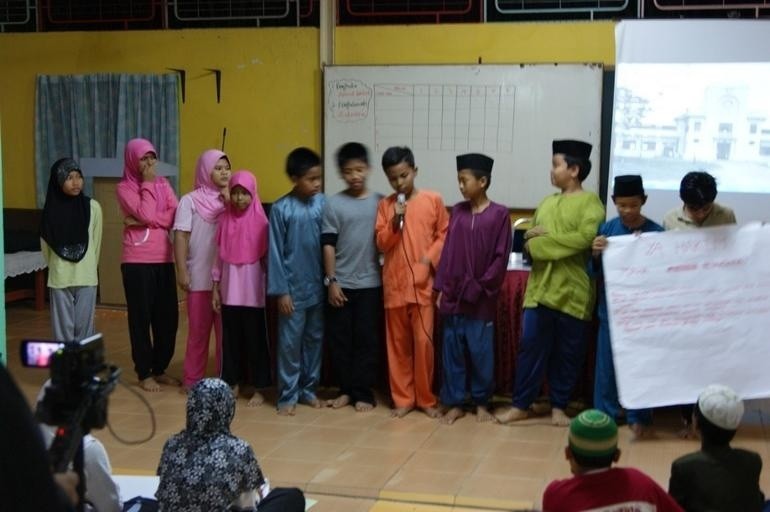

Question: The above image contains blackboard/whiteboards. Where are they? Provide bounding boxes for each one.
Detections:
[324,64,603,209]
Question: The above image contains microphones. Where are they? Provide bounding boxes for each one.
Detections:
[397,193,406,230]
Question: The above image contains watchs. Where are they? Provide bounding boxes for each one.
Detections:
[324,276,337,286]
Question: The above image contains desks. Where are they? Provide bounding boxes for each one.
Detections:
[3,250,49,312]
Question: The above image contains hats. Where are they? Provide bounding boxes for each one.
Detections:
[568,409,619,457]
[456,154,494,173]
[552,140,592,160]
[698,384,745,430]
[614,175,643,196]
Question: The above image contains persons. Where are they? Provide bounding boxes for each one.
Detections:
[0,359,79,511]
[666,384,766,512]
[33,370,126,512]
[267,147,332,416]
[173,148,233,393]
[114,136,180,394]
[153,375,306,510]
[432,153,512,425]
[374,146,449,419]
[591,175,666,437]
[321,141,387,412]
[210,170,270,412]
[541,407,685,511]
[491,139,607,425]
[664,168,739,439]
[40,156,105,342]
